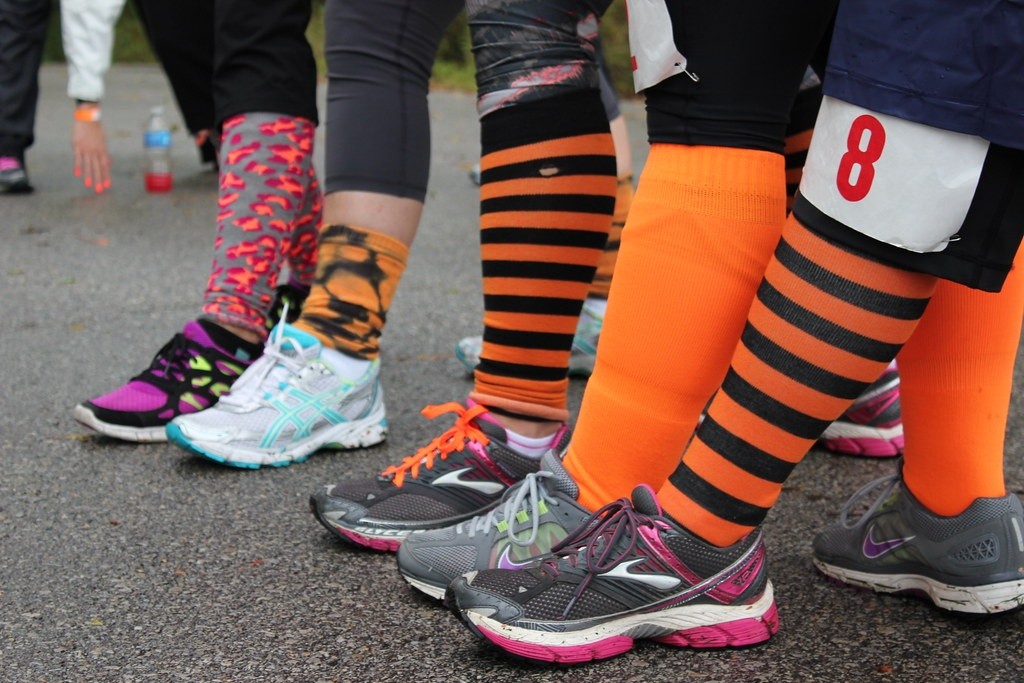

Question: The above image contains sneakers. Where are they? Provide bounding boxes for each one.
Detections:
[0,152,29,193]
[813,457,1024,613]
[265,274,310,342]
[445,485,780,663]
[309,398,571,550]
[398,449,606,600]
[455,303,602,376]
[819,357,904,456]
[74,321,259,442]
[165,302,389,468]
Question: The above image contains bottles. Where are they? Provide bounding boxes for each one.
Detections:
[144,107,172,191]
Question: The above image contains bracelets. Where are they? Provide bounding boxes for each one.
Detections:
[73,105,102,122]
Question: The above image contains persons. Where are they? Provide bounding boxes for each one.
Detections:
[304,1,908,554]
[161,0,643,472]
[443,1,1023,672]
[392,1,1023,622]
[0,0,224,198]
[72,0,326,447]
